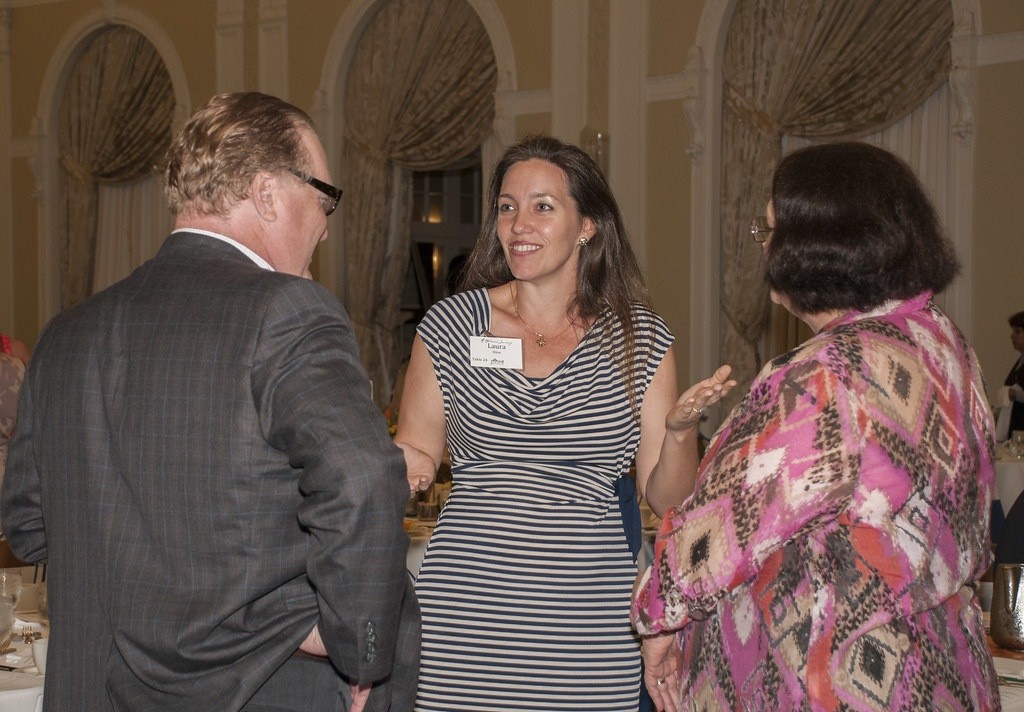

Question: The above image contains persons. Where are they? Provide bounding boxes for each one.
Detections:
[2,89,421,711]
[386,134,736,712]
[0,336,32,447]
[992,311,1024,443]
[627,142,1005,709]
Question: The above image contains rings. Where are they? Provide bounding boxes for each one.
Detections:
[694,407,705,413]
[656,680,665,685]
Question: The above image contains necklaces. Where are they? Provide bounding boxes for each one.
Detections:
[508,281,580,347]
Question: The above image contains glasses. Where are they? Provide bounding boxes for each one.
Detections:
[280,166,343,216]
